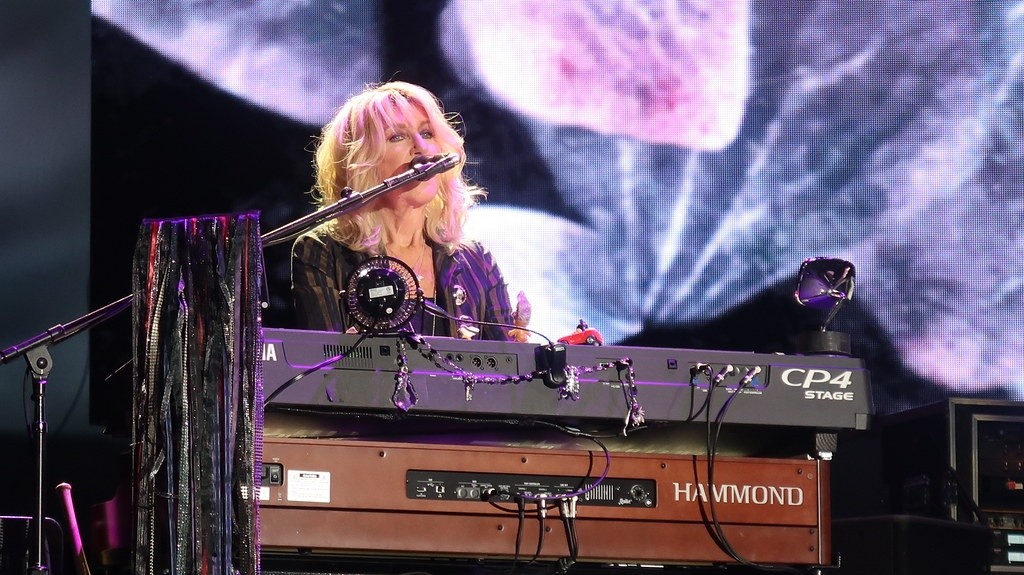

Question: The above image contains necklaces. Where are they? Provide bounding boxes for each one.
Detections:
[381,237,427,285]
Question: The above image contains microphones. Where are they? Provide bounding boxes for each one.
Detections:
[409,152,461,181]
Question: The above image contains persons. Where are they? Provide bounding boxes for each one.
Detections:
[288,79,529,342]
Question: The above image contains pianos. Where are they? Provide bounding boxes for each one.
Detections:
[244,253,880,575]
